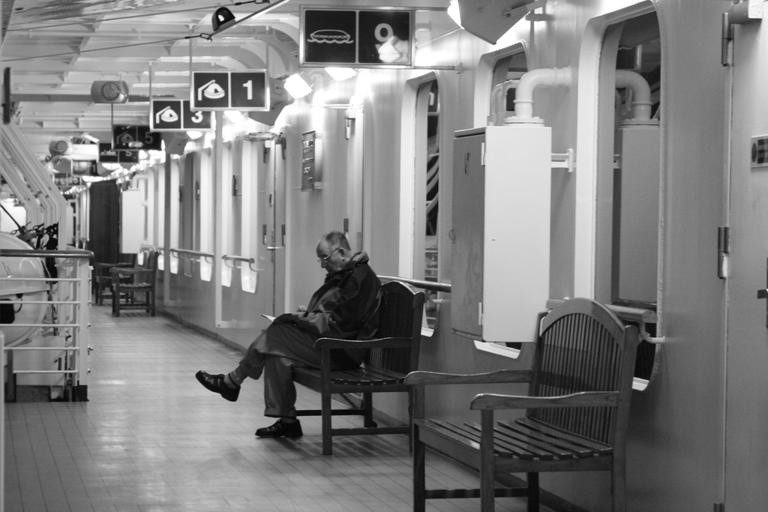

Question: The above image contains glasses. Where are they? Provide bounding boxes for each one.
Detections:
[315,246,343,264]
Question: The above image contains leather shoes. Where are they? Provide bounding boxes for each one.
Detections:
[254,416,305,440]
[195,370,241,403]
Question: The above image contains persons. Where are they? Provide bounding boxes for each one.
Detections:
[195,230,382,440]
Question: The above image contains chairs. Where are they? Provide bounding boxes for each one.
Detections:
[93,249,135,307]
[402,297,638,512]
[272,283,428,458]
[110,253,159,319]
